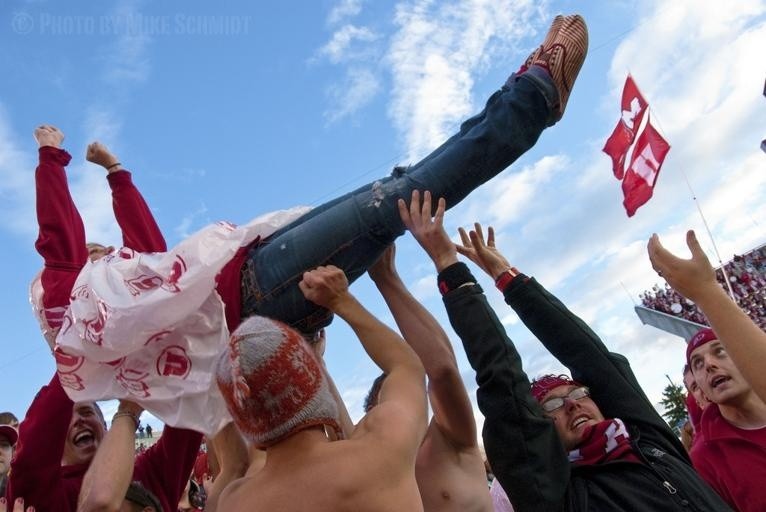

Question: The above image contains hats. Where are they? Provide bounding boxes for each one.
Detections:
[213,316,348,449]
[0,425,19,447]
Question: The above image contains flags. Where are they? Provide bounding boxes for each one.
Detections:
[621,112,670,218]
[601,71,649,181]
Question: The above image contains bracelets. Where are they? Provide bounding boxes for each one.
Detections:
[104,161,123,171]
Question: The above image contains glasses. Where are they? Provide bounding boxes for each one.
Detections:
[541,387,591,413]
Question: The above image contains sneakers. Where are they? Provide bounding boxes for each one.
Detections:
[536,15,589,122]
[522,14,564,70]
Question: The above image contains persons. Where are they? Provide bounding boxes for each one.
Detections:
[27,8,590,444]
[1,191,765,512]
